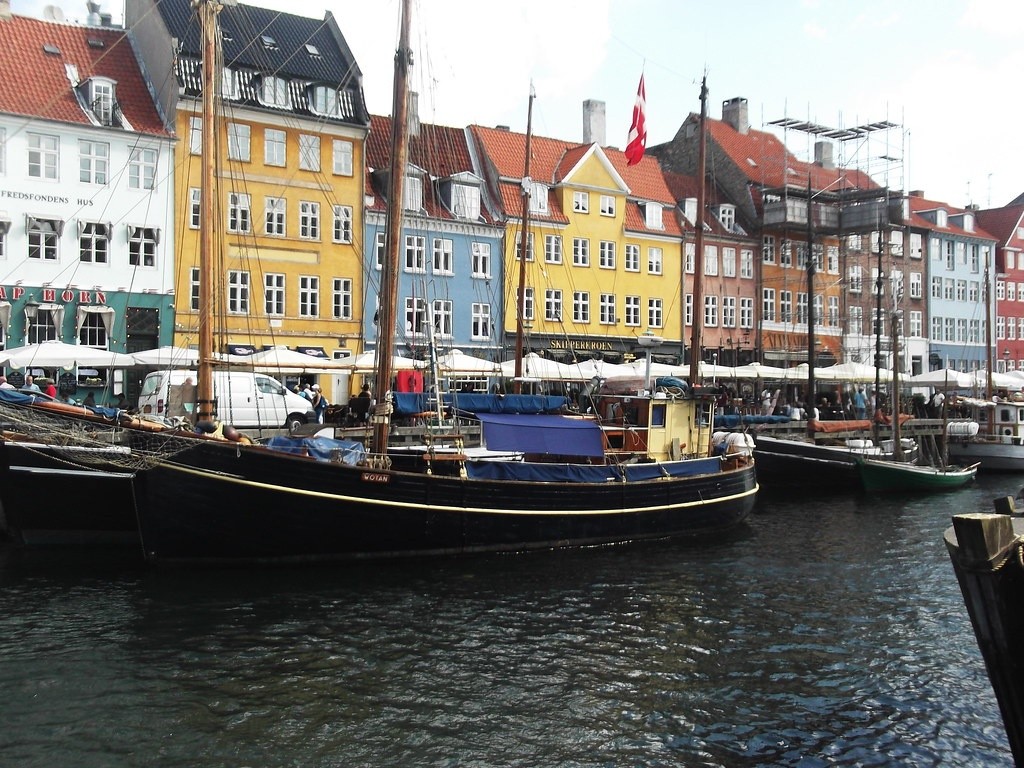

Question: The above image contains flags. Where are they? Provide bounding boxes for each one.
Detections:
[624,59,647,167]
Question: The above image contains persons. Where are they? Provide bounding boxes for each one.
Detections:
[713,385,731,416]
[761,385,1006,424]
[294,380,370,416]
[1,374,133,411]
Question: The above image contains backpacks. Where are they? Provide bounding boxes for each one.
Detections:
[303,391,312,403]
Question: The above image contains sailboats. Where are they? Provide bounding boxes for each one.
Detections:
[2,3,1022,580]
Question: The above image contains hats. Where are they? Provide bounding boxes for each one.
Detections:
[312,383,319,389]
[298,384,306,389]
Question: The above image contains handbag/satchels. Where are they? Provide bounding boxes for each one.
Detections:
[317,393,327,408]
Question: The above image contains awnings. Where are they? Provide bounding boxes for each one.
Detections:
[1,335,1023,395]
